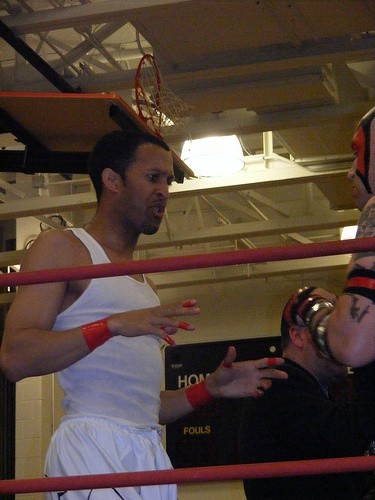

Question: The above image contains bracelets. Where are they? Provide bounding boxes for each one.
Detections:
[297,293,335,353]
[185,382,213,409]
[81,317,111,351]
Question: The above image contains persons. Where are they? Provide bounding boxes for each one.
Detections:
[281,101,375,366]
[2,127,287,500]
[236,286,375,500]
